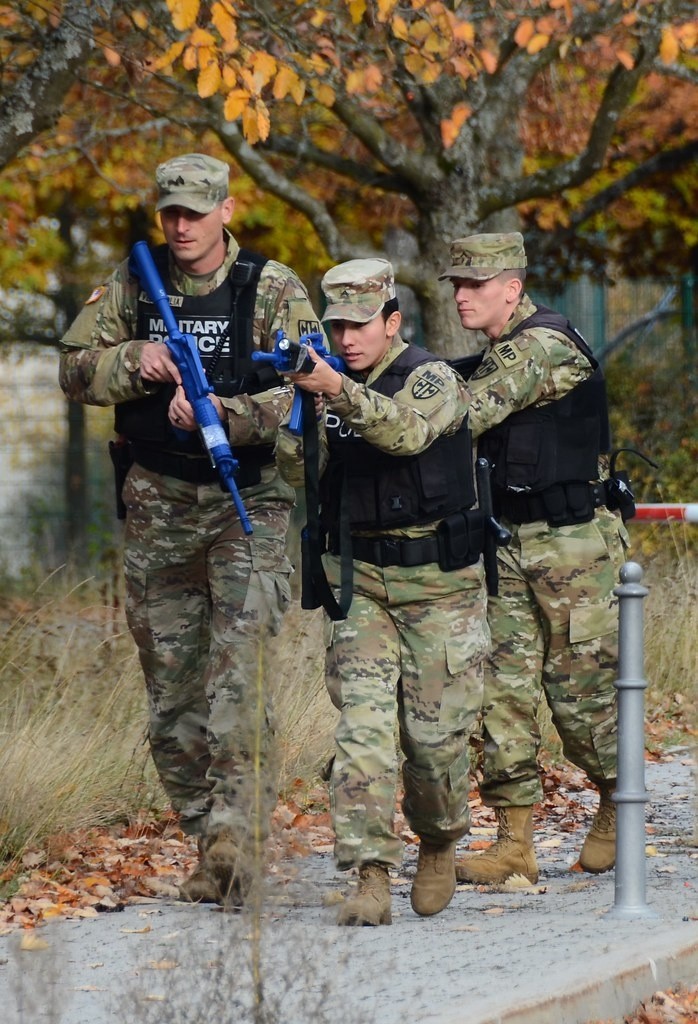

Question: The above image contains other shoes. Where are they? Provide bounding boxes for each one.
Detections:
[206,833,248,886]
[179,852,224,902]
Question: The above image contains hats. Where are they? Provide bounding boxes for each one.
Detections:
[437,232,528,282]
[155,152,230,214]
[320,257,396,323]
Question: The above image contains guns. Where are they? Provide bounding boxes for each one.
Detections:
[133,240,253,537]
[252,329,346,437]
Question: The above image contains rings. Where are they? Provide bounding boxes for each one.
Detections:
[176,416,182,422]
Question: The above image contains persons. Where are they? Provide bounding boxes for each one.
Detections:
[280,256,497,925]
[436,232,634,886]
[58,152,333,909]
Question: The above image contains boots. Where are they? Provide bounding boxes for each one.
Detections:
[455,805,539,885]
[338,865,392,926]
[579,782,616,874]
[410,839,456,916]
